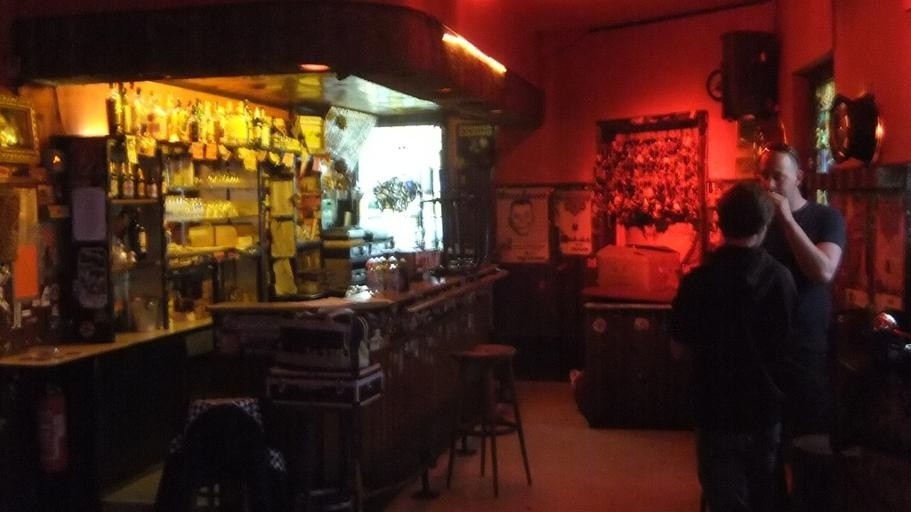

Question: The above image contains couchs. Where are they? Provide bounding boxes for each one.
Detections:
[568,295,696,428]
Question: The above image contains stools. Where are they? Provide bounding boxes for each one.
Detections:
[445,343,532,496]
[157,393,290,512]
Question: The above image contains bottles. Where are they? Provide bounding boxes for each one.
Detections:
[130,206,148,257]
[107,81,289,151]
[108,161,158,199]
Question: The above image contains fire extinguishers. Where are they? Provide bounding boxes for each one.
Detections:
[37,372,68,480]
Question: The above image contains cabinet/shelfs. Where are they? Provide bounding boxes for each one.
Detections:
[48,135,324,343]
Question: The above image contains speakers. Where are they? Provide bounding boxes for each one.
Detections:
[720,29,779,119]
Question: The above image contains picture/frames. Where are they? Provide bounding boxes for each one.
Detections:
[0,94,41,165]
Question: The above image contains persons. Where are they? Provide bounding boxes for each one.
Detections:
[756,137,848,511]
[667,180,800,512]
[507,200,537,247]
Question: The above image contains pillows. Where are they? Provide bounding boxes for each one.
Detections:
[579,287,676,304]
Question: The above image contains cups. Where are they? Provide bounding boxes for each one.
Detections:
[162,163,242,222]
